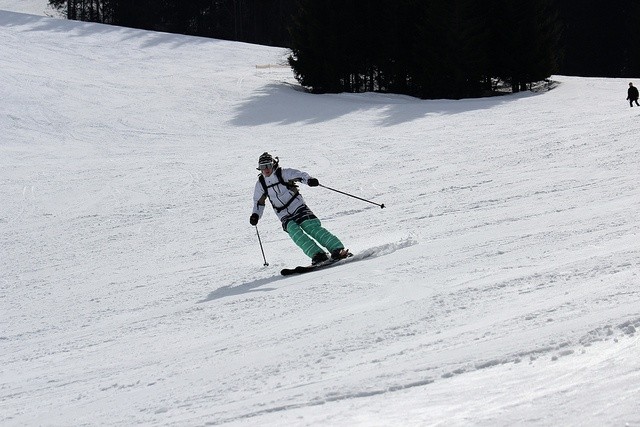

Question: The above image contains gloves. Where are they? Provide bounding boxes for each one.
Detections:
[250,213,259,225]
[307,179,319,187]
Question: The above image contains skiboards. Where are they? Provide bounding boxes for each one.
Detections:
[280,253,353,276]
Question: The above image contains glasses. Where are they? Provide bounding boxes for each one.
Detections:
[260,164,272,169]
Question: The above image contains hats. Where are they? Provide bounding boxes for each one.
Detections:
[258,152,274,169]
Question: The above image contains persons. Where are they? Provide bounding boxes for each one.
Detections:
[627,82,640,107]
[250,153,344,265]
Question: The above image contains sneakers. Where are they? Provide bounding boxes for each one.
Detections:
[311,252,329,265]
[331,249,347,259]
